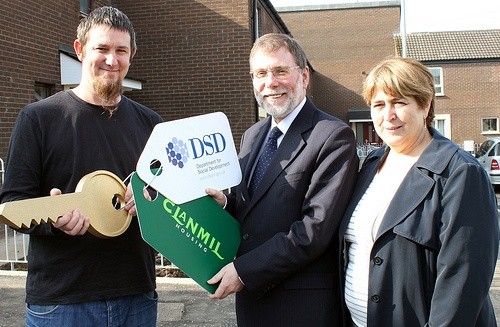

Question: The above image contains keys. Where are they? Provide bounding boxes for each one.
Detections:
[0,170,132,238]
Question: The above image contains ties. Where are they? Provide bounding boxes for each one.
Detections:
[248,126,283,200]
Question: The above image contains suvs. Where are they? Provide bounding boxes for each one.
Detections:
[473,137,500,191]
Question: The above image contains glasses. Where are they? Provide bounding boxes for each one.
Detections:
[249,65,299,80]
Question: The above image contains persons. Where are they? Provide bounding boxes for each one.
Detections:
[338,57,500,327]
[205,33,360,327]
[0,6,165,327]
